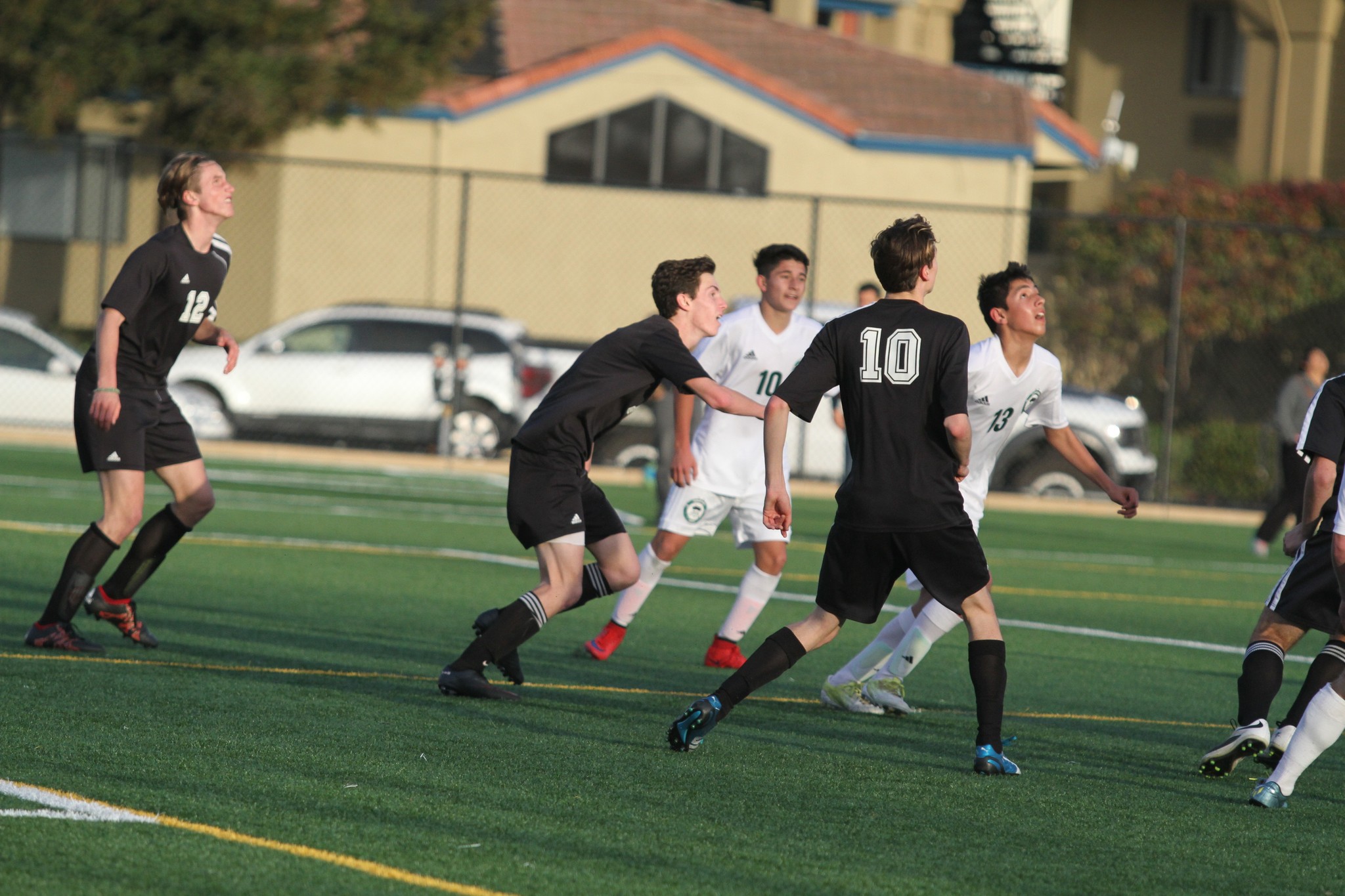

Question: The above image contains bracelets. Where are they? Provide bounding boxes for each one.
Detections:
[94,384,123,396]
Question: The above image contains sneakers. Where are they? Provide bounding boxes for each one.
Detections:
[1198,718,1270,780]
[862,677,914,719]
[1249,780,1287,810]
[438,665,522,704]
[83,585,158,649]
[26,621,105,654]
[704,634,747,669]
[585,621,626,661]
[472,608,524,685]
[973,736,1022,775]
[668,696,725,754]
[1254,721,1296,771]
[821,675,885,716]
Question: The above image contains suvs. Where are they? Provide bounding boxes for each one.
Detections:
[988,387,1159,502]
[165,296,560,465]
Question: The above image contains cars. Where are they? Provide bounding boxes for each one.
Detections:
[0,306,90,429]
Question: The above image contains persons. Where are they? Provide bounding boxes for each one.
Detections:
[583,243,840,669]
[23,153,240,654]
[832,282,881,431]
[1250,668,1345,809]
[821,260,1139,716]
[1197,373,1345,782]
[663,213,1023,777]
[1252,345,1331,558]
[437,254,766,701]
